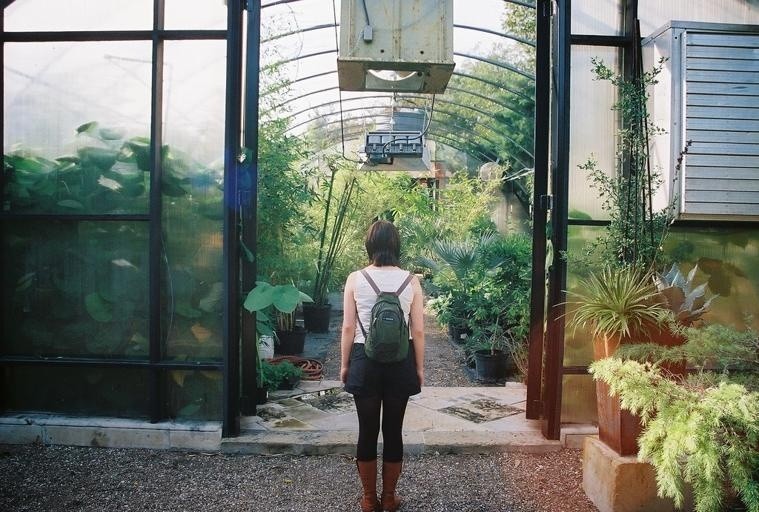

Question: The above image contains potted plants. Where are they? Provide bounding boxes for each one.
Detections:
[408,224,534,385]
[548,55,721,455]
[254,61,368,355]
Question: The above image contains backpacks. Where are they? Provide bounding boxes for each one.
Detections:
[355,269,414,363]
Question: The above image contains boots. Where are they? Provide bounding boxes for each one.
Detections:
[356,460,380,511]
[381,462,402,511]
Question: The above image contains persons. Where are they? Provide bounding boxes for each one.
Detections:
[339,219,427,512]
